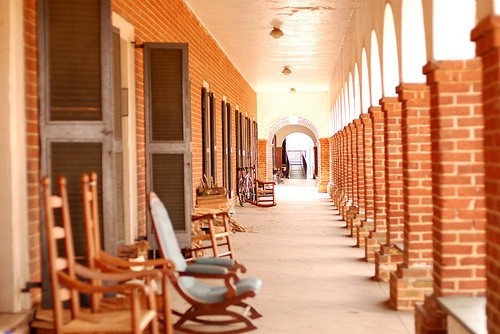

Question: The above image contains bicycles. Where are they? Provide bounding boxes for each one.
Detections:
[238,166,255,208]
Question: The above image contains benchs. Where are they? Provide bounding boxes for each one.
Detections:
[436,295,487,334]
[394,243,404,253]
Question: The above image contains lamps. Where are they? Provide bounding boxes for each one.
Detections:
[269,27,284,40]
[281,66,292,76]
[289,87,296,94]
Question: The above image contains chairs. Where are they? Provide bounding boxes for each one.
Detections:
[236,165,277,208]
[191,209,235,259]
[80,169,180,334]
[39,175,164,334]
[146,190,263,334]
